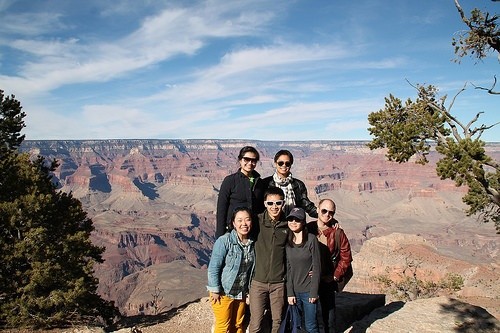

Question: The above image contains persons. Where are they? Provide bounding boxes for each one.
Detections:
[206,186,353,333]
[216,146,268,240]
[263,150,343,230]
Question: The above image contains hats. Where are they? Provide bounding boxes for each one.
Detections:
[286,208,306,219]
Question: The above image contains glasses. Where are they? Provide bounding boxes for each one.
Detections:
[321,209,334,215]
[277,161,290,167]
[242,156,258,162]
[264,200,284,208]
[288,217,302,222]
